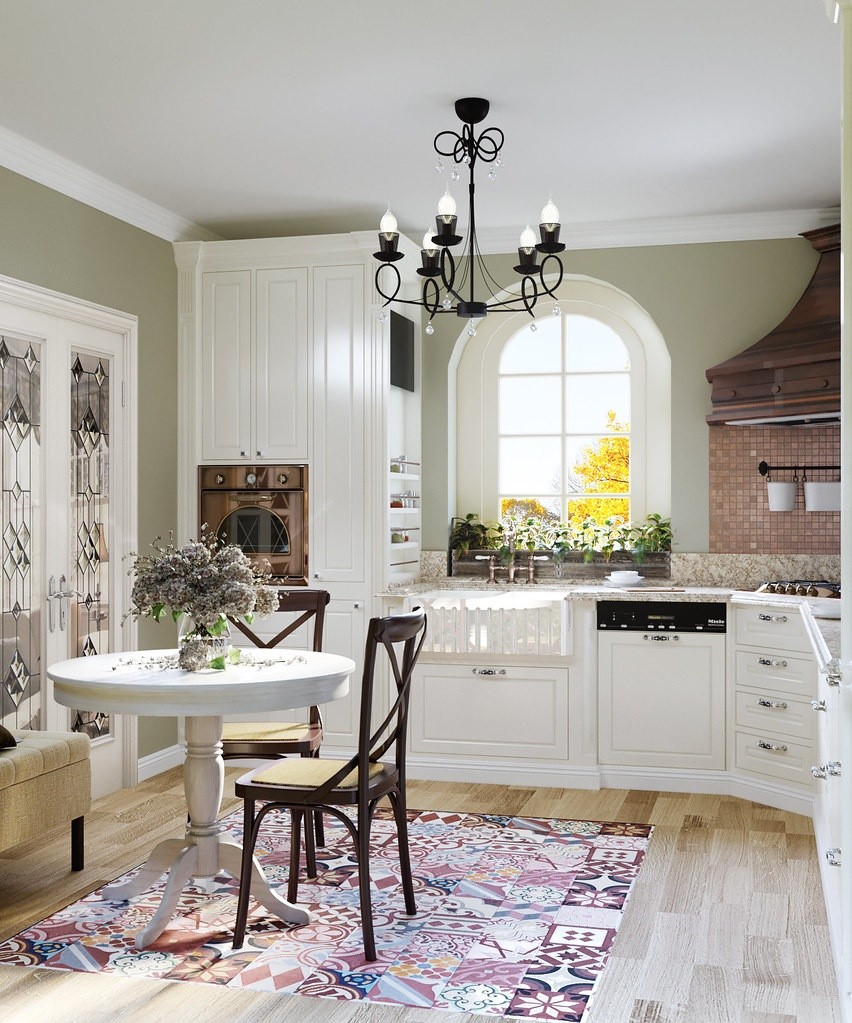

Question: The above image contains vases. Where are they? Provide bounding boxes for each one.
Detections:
[178,603,231,661]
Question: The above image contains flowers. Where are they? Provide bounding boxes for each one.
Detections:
[119,521,291,651]
[176,640,226,672]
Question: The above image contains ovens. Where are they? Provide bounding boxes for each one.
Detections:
[199,465,307,588]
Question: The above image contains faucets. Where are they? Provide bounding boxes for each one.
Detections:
[507,536,516,585]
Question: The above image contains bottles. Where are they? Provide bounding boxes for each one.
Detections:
[399,456,407,473]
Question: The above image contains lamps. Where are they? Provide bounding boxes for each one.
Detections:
[370,98,567,338]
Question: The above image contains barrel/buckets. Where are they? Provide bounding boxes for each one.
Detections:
[766,482,796,511]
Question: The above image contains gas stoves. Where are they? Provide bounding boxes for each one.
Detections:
[753,577,841,602]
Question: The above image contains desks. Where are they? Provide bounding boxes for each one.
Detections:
[46,648,354,950]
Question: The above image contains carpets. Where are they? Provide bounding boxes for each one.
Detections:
[0,799,654,1023]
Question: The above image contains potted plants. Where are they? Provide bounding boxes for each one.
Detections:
[448,512,673,578]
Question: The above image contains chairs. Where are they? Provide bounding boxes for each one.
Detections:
[188,589,331,879]
[232,608,417,963]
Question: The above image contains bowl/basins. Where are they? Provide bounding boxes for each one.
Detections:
[611,570,638,579]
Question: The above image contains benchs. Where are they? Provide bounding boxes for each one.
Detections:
[0,728,92,871]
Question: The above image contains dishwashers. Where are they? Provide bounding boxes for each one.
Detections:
[597,600,726,772]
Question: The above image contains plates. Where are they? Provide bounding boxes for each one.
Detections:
[605,575,646,584]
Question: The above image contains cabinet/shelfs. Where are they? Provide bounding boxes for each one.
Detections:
[383,597,602,790]
[598,631,731,796]
[172,228,421,769]
[727,602,842,1001]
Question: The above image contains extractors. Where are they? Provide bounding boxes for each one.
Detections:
[704,223,841,429]
